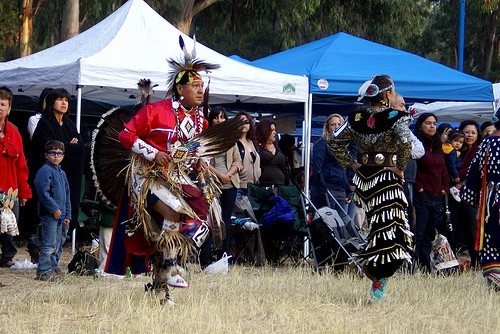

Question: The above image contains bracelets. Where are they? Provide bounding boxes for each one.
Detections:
[347,160,357,168]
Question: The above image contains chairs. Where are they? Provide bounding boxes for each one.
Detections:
[230,184,313,266]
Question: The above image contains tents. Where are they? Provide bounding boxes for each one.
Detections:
[0,0,311,261]
[227,31,494,266]
[408,82,500,123]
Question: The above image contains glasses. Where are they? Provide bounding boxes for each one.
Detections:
[47,151,63,157]
[425,121,437,126]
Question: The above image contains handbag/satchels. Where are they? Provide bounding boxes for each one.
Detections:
[68,232,101,277]
[203,252,232,277]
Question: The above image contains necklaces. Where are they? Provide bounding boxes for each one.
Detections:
[171,93,201,142]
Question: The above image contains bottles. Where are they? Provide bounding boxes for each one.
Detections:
[126,266,131,278]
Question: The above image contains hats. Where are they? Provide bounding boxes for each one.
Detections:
[162,35,221,99]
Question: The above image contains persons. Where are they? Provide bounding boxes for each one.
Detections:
[32,140,72,282]
[0,85,85,269]
[391,94,500,274]
[313,112,367,276]
[326,74,411,297]
[459,106,500,292]
[120,69,212,307]
[98,206,116,274]
[198,106,313,274]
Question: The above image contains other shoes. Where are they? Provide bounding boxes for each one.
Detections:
[167,269,189,288]
[2,260,15,268]
[38,267,65,282]
[450,186,461,202]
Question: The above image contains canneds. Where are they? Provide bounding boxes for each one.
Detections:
[126,267,132,278]
[94,269,100,281]
